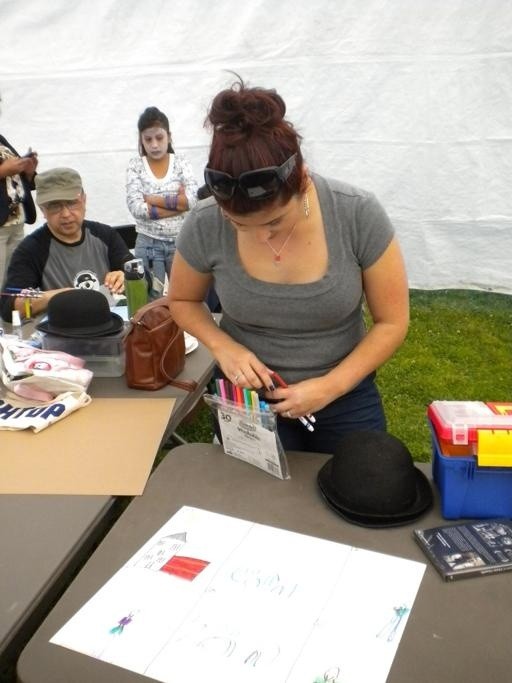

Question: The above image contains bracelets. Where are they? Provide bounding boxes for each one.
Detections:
[149,205,158,220]
[165,194,177,211]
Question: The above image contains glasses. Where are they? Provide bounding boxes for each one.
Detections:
[43,198,83,215]
[203,152,298,204]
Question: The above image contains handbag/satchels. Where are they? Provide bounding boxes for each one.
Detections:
[123,295,196,392]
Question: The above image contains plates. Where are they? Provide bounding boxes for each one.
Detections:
[184,330,199,355]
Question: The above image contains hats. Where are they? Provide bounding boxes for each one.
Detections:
[34,288,126,343]
[34,167,82,206]
[314,424,439,531]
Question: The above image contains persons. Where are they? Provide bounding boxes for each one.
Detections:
[0,167,151,324]
[125,107,201,302]
[167,68,410,456]
[0,135,38,288]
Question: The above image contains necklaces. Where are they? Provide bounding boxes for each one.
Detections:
[265,225,297,262]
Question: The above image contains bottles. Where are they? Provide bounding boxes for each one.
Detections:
[124,258,149,321]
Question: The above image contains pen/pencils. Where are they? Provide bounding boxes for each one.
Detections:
[216,379,274,428]
[267,369,316,432]
[1,288,47,299]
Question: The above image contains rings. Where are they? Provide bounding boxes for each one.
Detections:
[235,373,242,380]
[286,410,292,419]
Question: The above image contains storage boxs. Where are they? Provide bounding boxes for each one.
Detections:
[422,398,510,521]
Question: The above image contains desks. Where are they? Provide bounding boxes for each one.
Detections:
[0,306,229,659]
[8,437,510,683]
